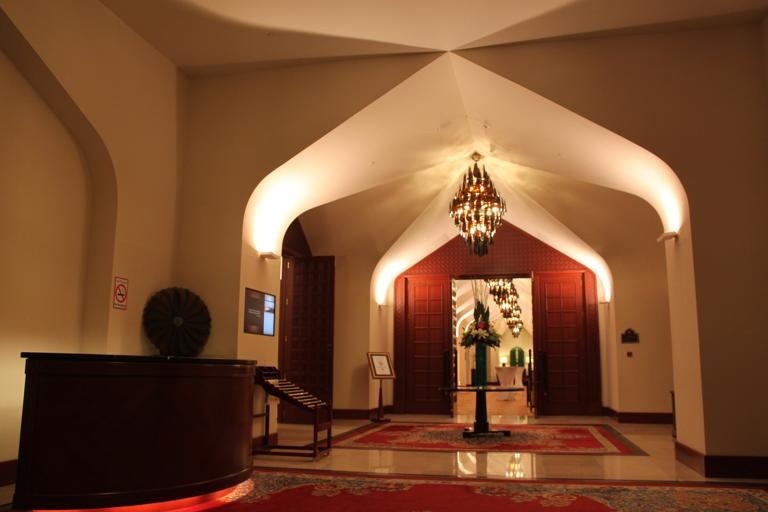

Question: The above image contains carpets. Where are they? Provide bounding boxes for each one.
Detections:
[0,463,768,512]
[306,419,651,456]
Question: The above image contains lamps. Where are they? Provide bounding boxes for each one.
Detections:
[447,152,508,257]
[484,277,524,338]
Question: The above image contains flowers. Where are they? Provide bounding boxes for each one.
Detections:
[459,299,502,351]
